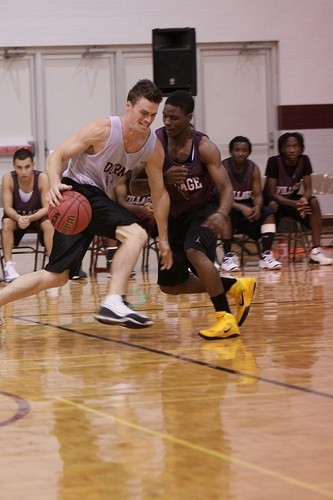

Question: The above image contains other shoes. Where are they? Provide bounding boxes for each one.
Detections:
[71,271,87,280]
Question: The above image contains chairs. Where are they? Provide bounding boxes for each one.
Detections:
[0,175,313,282]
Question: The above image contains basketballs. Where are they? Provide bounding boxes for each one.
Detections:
[48,190,92,235]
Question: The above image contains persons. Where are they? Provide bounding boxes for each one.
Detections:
[0,78,256,340]
[220,136,282,272]
[263,131,333,269]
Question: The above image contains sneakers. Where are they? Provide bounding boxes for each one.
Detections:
[106,260,136,277]
[258,249,283,269]
[94,294,154,329]
[220,252,241,272]
[306,247,333,266]
[226,277,256,327]
[198,311,240,340]
[3,262,20,283]
